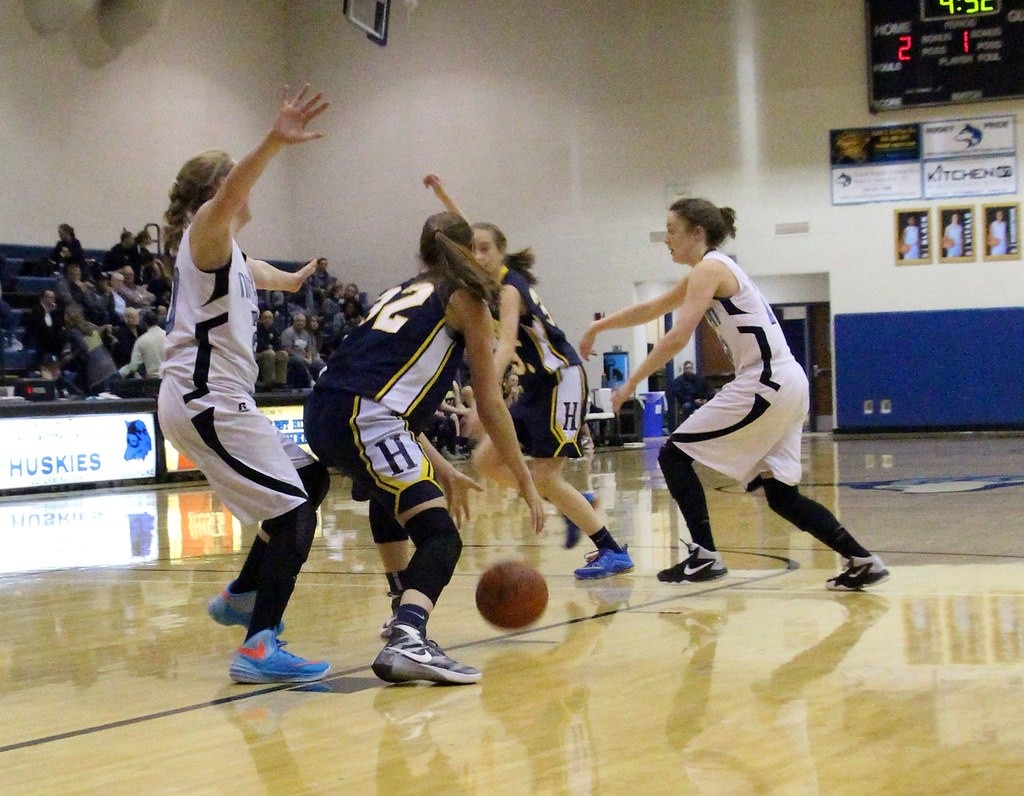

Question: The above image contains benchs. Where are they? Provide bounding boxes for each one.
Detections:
[0,243,299,402]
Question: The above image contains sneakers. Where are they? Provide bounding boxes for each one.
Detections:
[206,580,284,635]
[658,538,729,584]
[562,491,601,548]
[574,544,635,580]
[371,623,481,684]
[826,552,890,590]
[380,590,404,643]
[229,624,331,683]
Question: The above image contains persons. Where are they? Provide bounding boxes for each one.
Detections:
[303,211,545,685]
[673,361,707,420]
[988,210,1009,255]
[424,173,636,580]
[942,213,964,257]
[157,84,331,684]
[899,216,922,259]
[0,224,524,462]
[579,198,890,590]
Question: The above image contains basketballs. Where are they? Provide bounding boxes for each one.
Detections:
[474,561,550,629]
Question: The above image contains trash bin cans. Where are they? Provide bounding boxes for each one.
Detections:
[640,390,668,440]
